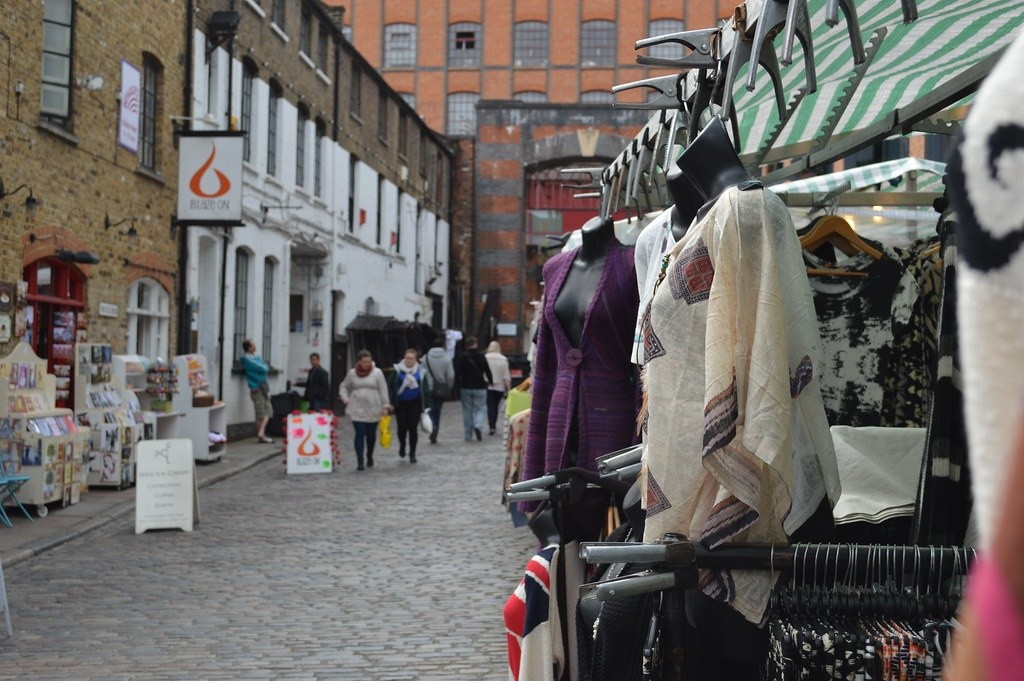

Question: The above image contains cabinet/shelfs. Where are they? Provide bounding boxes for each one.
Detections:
[173,353,228,461]
[0,341,91,506]
[75,343,144,489]
[112,355,186,441]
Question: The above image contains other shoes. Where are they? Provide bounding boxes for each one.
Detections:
[399,444,406,457]
[474,427,482,442]
[489,429,494,434]
[367,457,373,467]
[357,464,364,470]
[410,454,416,463]
[429,434,438,444]
[258,438,275,443]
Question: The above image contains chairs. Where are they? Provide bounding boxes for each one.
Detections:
[0,457,33,528]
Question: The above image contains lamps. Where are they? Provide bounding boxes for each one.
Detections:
[0,174,39,205]
[171,113,221,129]
[105,212,137,236]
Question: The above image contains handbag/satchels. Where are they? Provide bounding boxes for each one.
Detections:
[431,380,448,401]
[420,407,434,434]
[378,413,392,449]
[260,381,269,396]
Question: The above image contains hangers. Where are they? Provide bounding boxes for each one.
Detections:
[516,377,532,393]
[765,537,987,651]
[921,235,943,272]
[799,191,882,278]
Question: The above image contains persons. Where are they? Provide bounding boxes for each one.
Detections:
[943,22,1022,681]
[390,349,431,464]
[422,336,510,441]
[517,215,667,546]
[240,338,282,443]
[340,350,391,471]
[637,117,839,623]
[303,351,329,412]
